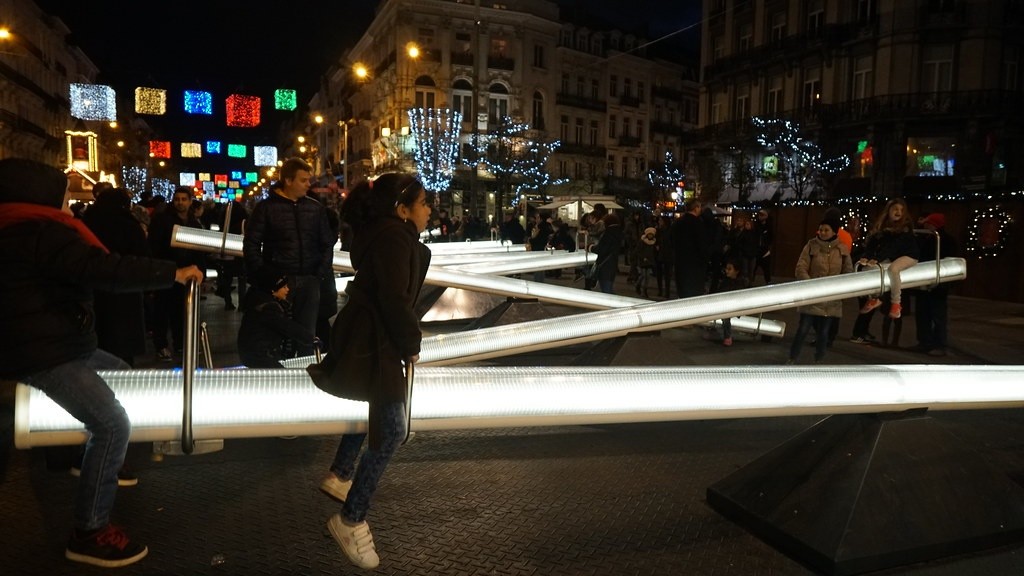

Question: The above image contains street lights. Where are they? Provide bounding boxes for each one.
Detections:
[315,115,347,192]
[298,136,321,175]
[100,117,125,172]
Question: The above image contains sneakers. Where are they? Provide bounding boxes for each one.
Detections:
[328,512,379,569]
[320,474,353,503]
[175,346,203,355]
[63,523,148,567]
[72,454,139,486]
[850,336,871,344]
[155,349,172,361]
[861,298,882,313]
[888,304,902,318]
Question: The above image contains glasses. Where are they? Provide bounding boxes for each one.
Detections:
[394,172,422,208]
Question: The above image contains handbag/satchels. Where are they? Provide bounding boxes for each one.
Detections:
[590,262,600,288]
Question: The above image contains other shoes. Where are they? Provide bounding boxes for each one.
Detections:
[574,273,583,283]
[722,337,732,345]
[703,331,720,340]
[785,358,795,365]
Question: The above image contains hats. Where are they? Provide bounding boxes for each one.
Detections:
[926,213,944,228]
[645,228,657,236]
[248,262,287,297]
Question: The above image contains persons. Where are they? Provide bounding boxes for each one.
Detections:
[785,198,956,366]
[428,201,770,346]
[0,157,345,568]
[309,174,430,568]
